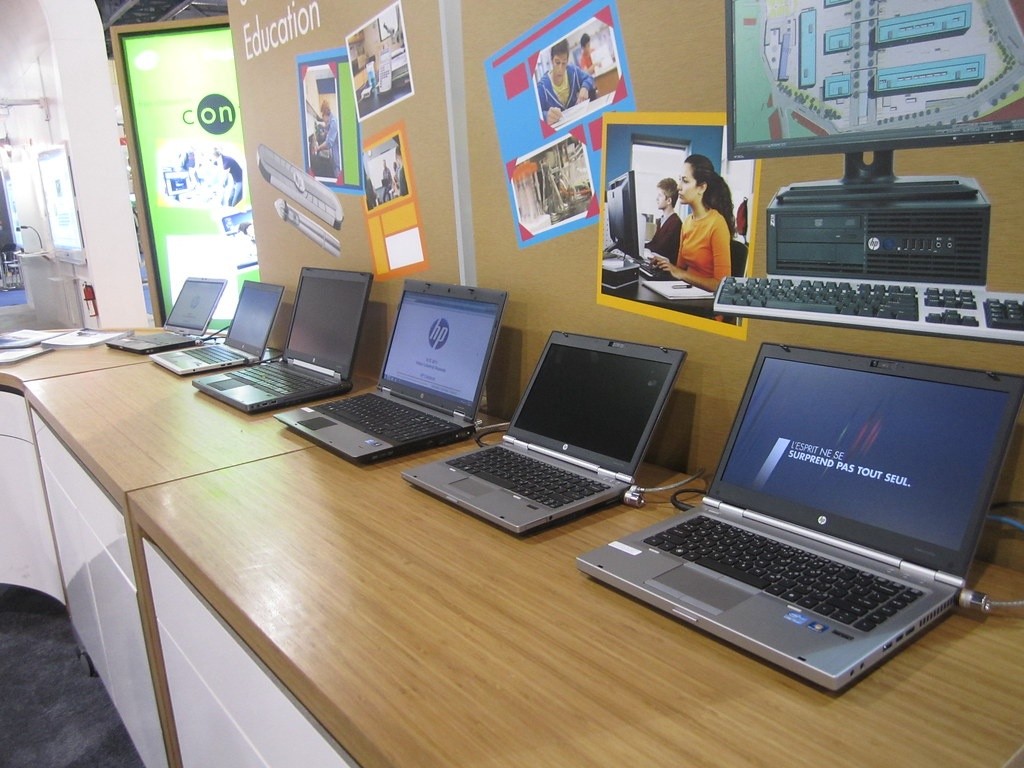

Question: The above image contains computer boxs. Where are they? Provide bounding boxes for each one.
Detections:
[762,176,991,288]
[601,259,639,290]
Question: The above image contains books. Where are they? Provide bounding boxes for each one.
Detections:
[0,327,134,362]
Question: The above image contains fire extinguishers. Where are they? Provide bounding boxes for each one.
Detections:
[81,280,99,317]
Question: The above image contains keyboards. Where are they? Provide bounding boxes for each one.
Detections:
[638,263,671,280]
[714,275,1024,342]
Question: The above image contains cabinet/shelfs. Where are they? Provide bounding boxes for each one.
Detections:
[1,327,228,606]
[126,410,1024,768]
[20,355,379,768]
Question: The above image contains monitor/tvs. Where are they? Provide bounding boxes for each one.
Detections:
[604,171,641,262]
[722,1,1023,201]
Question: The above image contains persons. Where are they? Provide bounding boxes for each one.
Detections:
[578,33,604,75]
[642,151,733,292]
[536,38,595,126]
[309,100,340,178]
[363,145,408,211]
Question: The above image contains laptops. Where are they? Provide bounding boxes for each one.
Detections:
[105,266,1024,692]
